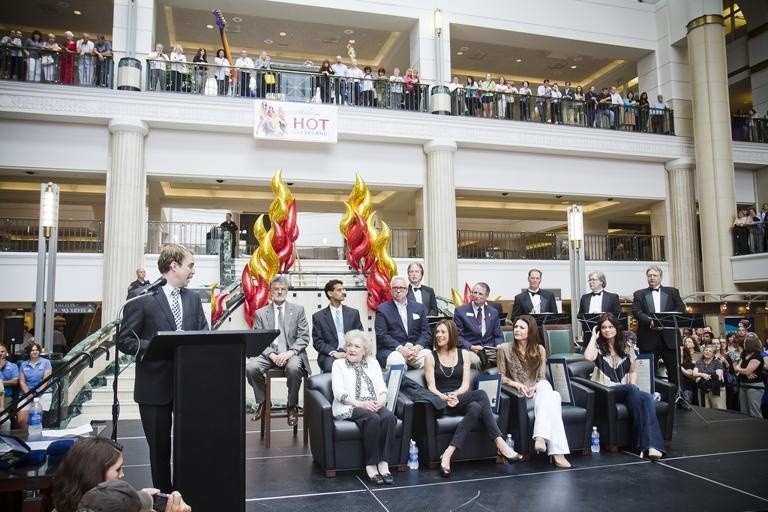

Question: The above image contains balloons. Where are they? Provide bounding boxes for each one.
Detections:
[208,170,401,326]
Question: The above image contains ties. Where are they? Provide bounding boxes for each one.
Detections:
[277,305,288,355]
[478,307,482,335]
[171,286,182,329]
[335,310,345,332]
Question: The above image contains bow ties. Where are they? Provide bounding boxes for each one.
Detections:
[650,287,659,292]
[414,287,421,291]
[531,290,540,296]
[592,292,601,296]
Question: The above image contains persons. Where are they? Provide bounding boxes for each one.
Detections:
[48,436,125,511]
[537,79,668,133]
[127,266,151,292]
[450,73,532,121]
[76,476,193,511]
[118,244,213,493]
[319,56,421,110]
[16,342,53,430]
[2,30,112,87]
[147,43,272,99]
[247,263,686,486]
[679,317,768,419]
[609,229,652,261]
[0,344,20,419]
[733,201,767,255]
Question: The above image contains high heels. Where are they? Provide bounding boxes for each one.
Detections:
[639,449,663,461]
[550,455,572,468]
[496,448,525,465]
[439,454,451,478]
[535,442,547,454]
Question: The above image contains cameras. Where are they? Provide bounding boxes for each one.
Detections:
[152,494,172,512]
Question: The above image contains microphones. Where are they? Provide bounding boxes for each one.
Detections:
[140,278,167,295]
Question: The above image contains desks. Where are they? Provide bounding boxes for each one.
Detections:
[0,424,109,512]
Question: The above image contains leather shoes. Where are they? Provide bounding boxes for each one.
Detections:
[252,401,272,420]
[361,464,394,484]
[286,405,297,426]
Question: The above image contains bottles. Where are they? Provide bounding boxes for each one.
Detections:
[28,397,42,439]
[591,425,601,455]
[506,433,515,450]
[409,440,420,470]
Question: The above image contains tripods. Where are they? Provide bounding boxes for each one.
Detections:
[674,325,712,428]
